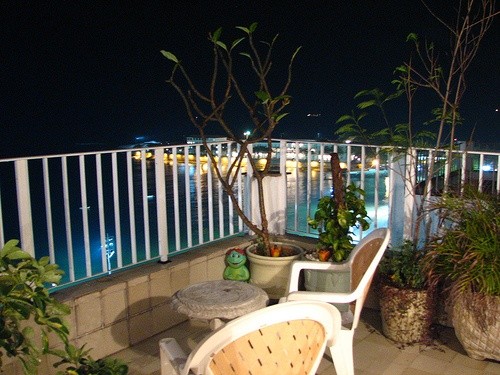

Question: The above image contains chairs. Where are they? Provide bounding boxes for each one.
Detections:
[278,228,391,375]
[158,300,342,375]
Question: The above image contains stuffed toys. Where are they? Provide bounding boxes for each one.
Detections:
[223,248,250,281]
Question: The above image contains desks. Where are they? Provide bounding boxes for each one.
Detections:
[171,280,268,331]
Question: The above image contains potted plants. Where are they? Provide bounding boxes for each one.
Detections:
[415,169,500,362]
[334,0,500,344]
[303,153,372,325]
[158,22,304,301]
